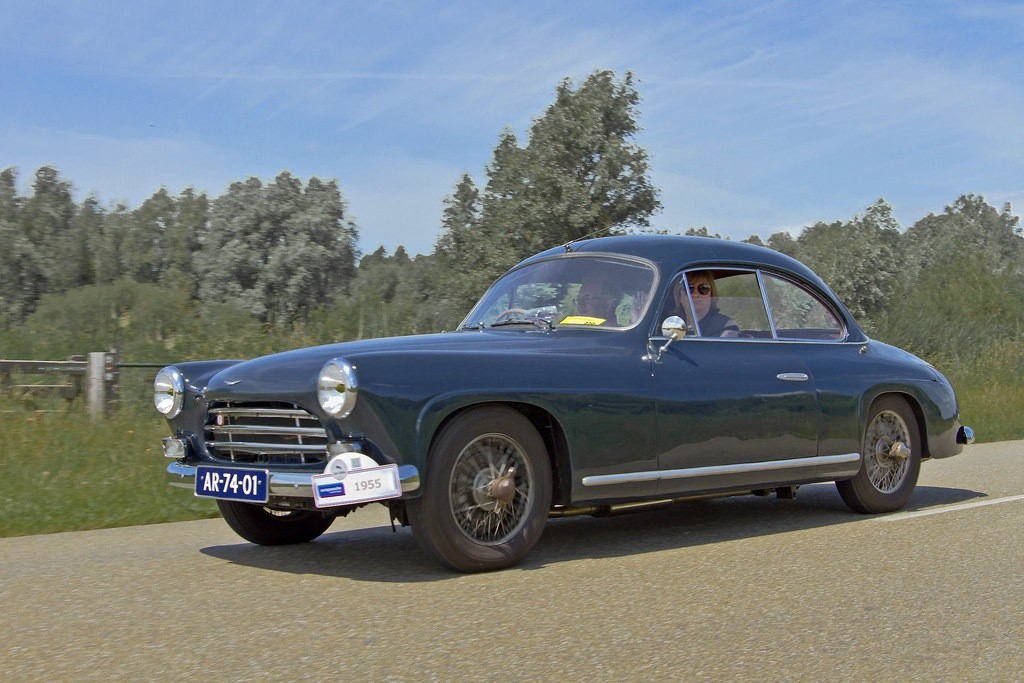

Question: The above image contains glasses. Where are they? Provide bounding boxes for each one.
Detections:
[572,296,604,306]
[682,285,711,295]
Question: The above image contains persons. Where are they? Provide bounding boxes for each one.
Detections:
[631,269,740,338]
[573,274,623,327]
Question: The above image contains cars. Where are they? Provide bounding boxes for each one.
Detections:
[153,231,976,575]
